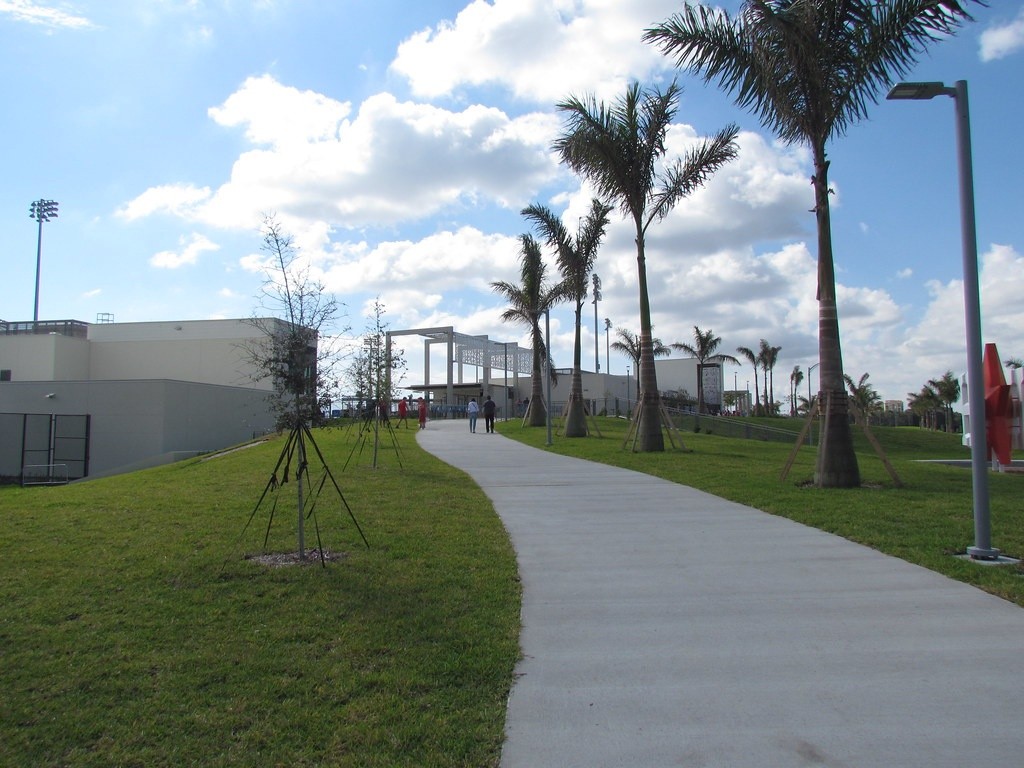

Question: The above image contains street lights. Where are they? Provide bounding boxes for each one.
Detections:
[626,366,629,419]
[495,342,508,422]
[364,339,372,398]
[531,308,554,445]
[605,318,611,374]
[592,274,602,372]
[746,381,749,414]
[887,80,1020,566]
[29,199,59,320]
[735,372,737,410]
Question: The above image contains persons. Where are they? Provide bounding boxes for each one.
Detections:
[515,399,522,418]
[523,397,530,417]
[483,395,495,433]
[396,397,409,429]
[712,409,729,417]
[418,397,426,429]
[468,398,479,433]
